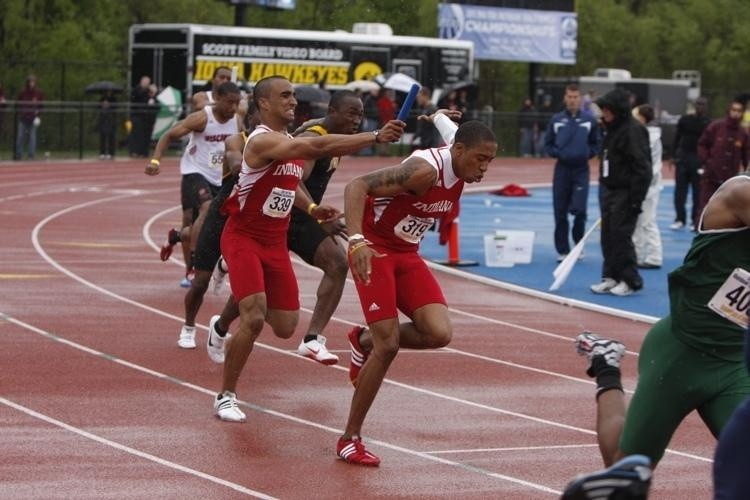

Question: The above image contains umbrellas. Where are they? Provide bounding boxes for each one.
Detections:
[151,86,182,141]
[86,82,122,93]
[372,72,422,93]
[437,81,478,106]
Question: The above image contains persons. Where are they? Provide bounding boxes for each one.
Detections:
[336,109,497,466]
[192,66,246,116]
[695,100,750,230]
[213,75,406,423]
[544,85,602,263]
[574,174,750,470]
[207,90,364,364]
[13,75,44,159]
[177,101,260,348]
[96,91,117,160]
[558,397,750,500]
[590,88,653,296]
[534,94,554,158]
[631,106,663,267]
[144,81,243,287]
[355,87,467,149]
[669,98,711,231]
[128,76,158,159]
[518,98,536,157]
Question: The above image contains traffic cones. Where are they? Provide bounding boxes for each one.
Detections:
[437,217,477,266]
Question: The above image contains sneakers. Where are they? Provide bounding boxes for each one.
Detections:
[559,455,651,500]
[575,331,626,377]
[578,250,584,260]
[336,435,380,466]
[557,254,566,261]
[207,314,232,364]
[186,264,195,280]
[347,326,371,388]
[177,325,196,348]
[160,228,177,261]
[610,279,643,297]
[210,259,224,296]
[590,276,617,294]
[669,220,683,230]
[296,334,339,365]
[213,389,246,422]
